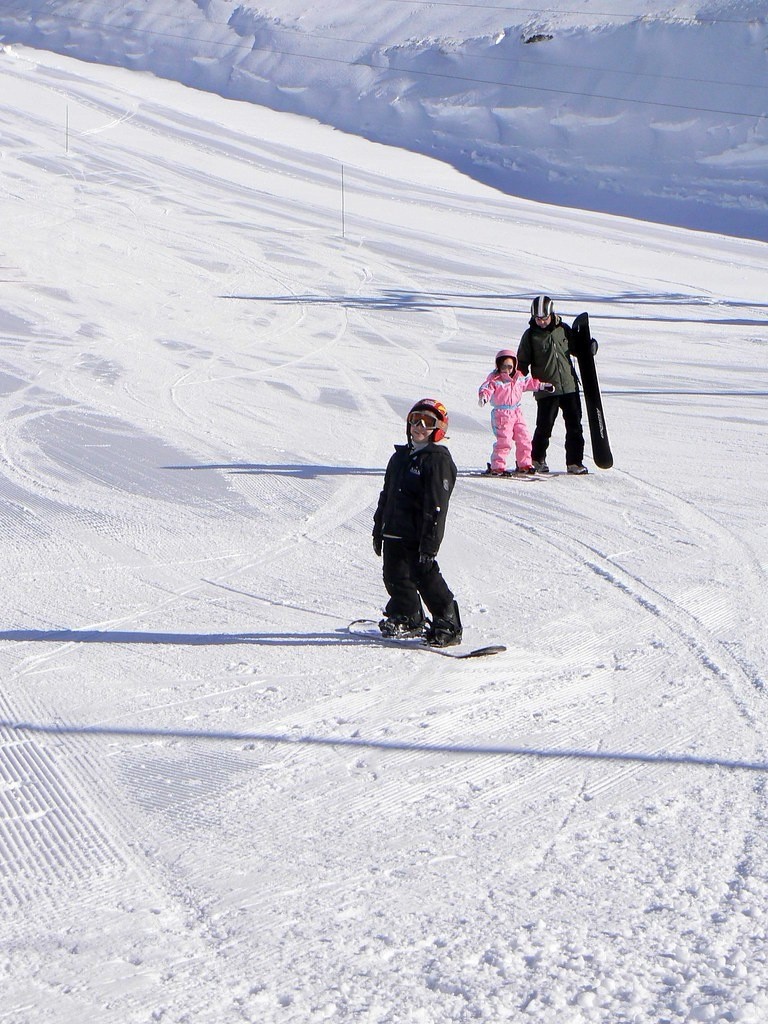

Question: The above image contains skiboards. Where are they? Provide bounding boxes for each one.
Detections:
[468,464,564,483]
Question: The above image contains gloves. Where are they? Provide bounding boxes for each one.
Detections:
[589,338,598,355]
[416,553,435,575]
[540,382,556,394]
[478,395,489,407]
[373,535,384,556]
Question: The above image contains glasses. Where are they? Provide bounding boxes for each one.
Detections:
[499,365,514,370]
[535,316,550,320]
[408,411,441,431]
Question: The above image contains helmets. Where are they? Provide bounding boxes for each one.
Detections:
[495,350,518,371]
[530,295,554,317]
[406,398,449,447]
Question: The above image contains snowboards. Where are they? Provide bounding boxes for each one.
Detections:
[350,618,507,660]
[571,309,616,470]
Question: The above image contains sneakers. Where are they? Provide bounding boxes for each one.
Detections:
[421,615,463,648]
[515,461,537,475]
[567,465,588,475]
[378,614,426,639]
[485,463,513,477]
[532,459,550,474]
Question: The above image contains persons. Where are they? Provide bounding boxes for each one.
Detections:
[372,398,462,647]
[478,350,555,477]
[517,295,598,475]
